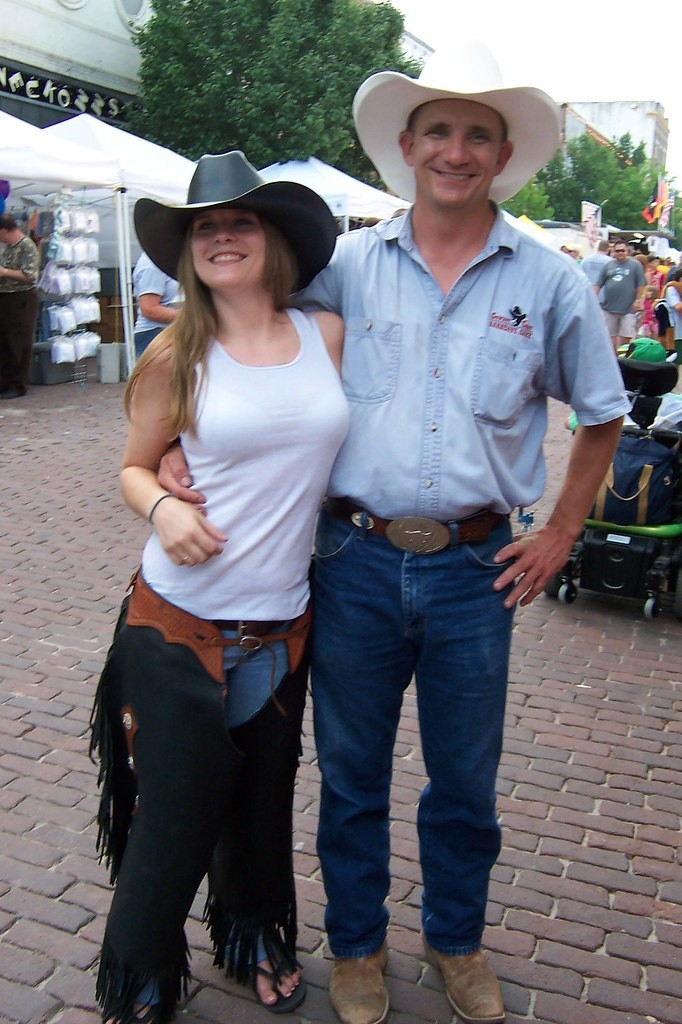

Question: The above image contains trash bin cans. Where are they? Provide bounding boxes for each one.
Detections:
[98,344,121,383]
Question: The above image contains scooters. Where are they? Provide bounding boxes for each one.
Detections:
[538,347,681,623]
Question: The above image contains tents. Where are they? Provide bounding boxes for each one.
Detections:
[0,109,563,378]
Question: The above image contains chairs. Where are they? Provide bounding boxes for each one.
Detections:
[616,356,682,458]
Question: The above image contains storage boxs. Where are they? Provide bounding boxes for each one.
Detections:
[27,342,75,385]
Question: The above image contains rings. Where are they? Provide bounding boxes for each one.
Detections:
[184,555,189,560]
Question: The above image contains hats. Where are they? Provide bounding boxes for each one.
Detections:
[133,151,336,293]
[617,337,667,363]
[351,51,562,205]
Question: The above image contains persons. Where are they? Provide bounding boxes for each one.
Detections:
[561,238,682,363]
[87,149,346,1024]
[157,42,632,1024]
[564,336,682,433]
[0,212,41,399]
[132,251,182,359]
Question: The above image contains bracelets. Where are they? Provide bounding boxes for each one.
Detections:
[149,494,172,525]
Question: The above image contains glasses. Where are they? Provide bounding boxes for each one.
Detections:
[615,249,627,254]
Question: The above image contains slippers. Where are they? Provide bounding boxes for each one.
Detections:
[102,1003,176,1024]
[254,958,307,1013]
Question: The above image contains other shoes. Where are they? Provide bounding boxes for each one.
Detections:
[0,385,26,400]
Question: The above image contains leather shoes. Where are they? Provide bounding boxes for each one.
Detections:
[422,932,507,1022]
[330,945,391,1023]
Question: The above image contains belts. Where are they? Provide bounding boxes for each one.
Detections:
[345,508,502,554]
[218,619,278,635]
